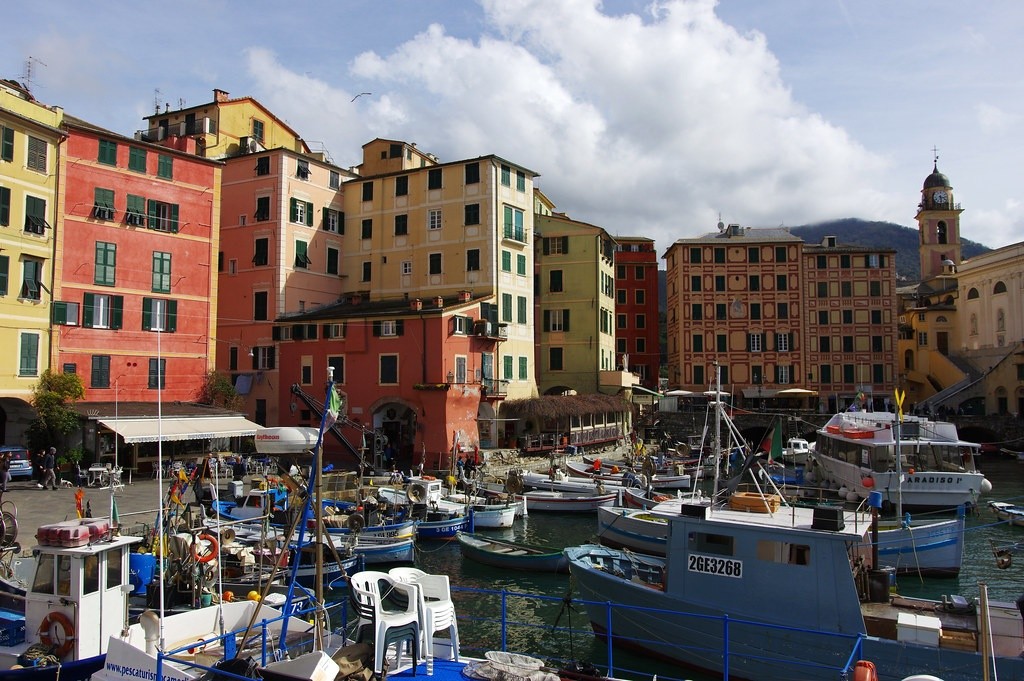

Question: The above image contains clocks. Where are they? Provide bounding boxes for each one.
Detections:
[934,190,948,203]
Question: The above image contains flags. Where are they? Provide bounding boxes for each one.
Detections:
[760,419,784,465]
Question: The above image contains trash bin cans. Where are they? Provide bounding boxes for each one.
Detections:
[572,447,577,456]
[302,465,312,481]
[568,445,573,453]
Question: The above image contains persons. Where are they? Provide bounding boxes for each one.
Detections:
[887,401,965,416]
[457,455,476,480]
[223,456,247,481]
[0,446,60,492]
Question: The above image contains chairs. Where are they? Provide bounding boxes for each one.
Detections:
[348,567,460,681]
[151,461,182,480]
[78,463,123,487]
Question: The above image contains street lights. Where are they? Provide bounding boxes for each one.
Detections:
[115,373,127,471]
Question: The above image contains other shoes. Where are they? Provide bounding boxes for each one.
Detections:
[52,486,58,490]
[3,490,10,492]
[42,486,49,490]
[36,483,43,487]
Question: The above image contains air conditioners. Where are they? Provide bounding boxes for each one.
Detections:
[239,137,266,155]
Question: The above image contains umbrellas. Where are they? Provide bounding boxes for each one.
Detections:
[776,388,818,397]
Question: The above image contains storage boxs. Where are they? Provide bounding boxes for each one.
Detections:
[896,612,977,652]
[37,516,111,547]
[251,551,288,567]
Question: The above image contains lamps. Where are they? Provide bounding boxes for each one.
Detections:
[247,347,254,357]
[446,371,454,384]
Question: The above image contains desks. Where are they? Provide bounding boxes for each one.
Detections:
[88,468,107,486]
[123,467,138,485]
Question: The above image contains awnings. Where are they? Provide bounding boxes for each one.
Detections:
[96,416,266,444]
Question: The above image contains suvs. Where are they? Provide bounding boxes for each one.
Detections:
[0,446,34,481]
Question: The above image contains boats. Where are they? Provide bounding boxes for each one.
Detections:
[598,349,883,601]
[0,364,816,680]
[804,409,993,517]
[562,496,1024,680]
[986,499,1024,528]
